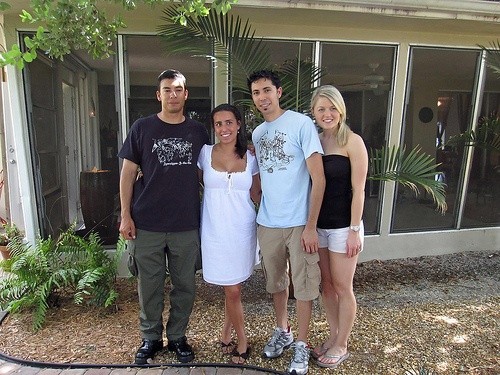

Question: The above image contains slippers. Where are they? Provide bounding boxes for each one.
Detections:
[312,343,329,358]
[220,341,236,354]
[232,347,249,365]
[317,352,350,368]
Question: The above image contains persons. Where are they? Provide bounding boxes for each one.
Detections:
[136,103,263,365]
[247,69,326,375]
[116,68,255,364]
[309,85,368,368]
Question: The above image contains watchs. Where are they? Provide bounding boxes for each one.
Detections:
[350,225,360,232]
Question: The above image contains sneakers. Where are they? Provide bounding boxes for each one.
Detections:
[263,327,294,358]
[288,341,310,375]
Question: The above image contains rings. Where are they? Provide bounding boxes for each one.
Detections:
[357,249,360,253]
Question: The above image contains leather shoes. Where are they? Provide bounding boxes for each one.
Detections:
[168,337,193,363]
[135,339,163,363]
